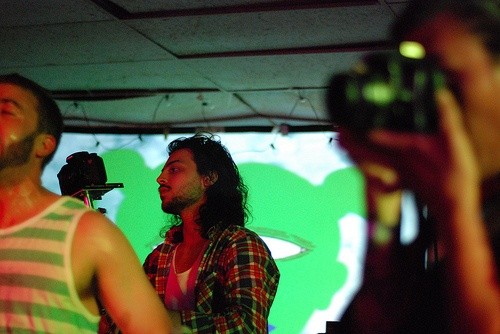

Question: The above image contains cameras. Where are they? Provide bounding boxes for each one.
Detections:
[326,48,463,138]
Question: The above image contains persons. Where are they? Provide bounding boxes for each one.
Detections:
[0,73,175,334]
[324,0,500,334]
[114,131,280,334]
